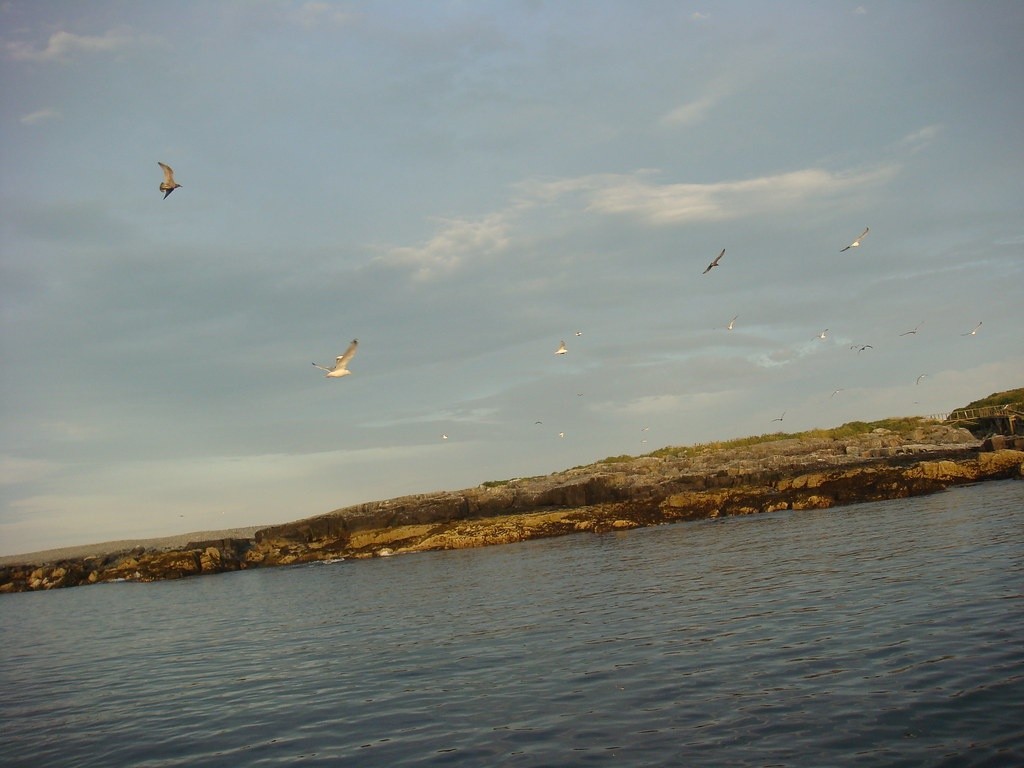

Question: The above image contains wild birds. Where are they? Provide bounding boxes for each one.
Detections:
[849,344,873,352]
[640,439,648,443]
[641,426,650,432]
[559,431,564,439]
[703,248,725,274]
[574,329,582,336]
[726,316,739,331]
[899,320,925,337]
[534,420,543,425]
[811,328,832,343]
[915,373,928,385]
[439,432,449,441]
[914,401,920,404]
[577,393,584,396]
[839,228,870,252]
[553,339,568,356]
[960,321,983,336]
[830,388,844,399]
[155,161,184,199]
[768,408,788,424]
[1002,403,1013,410]
[312,338,361,380]
